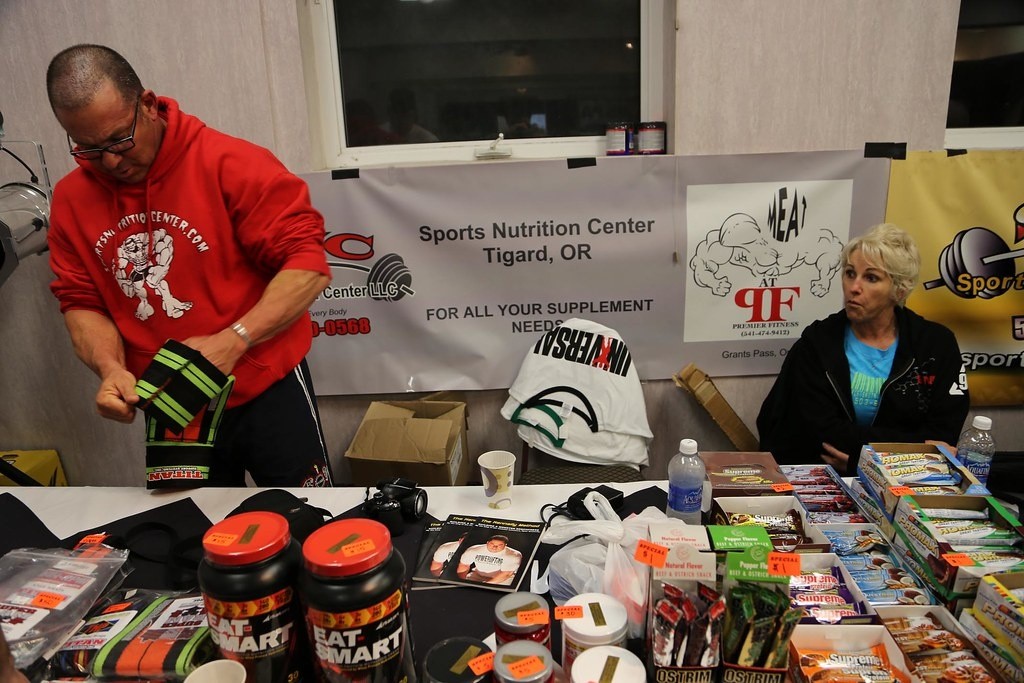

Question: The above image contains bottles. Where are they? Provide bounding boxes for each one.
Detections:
[956,416,995,487]
[666,438,705,526]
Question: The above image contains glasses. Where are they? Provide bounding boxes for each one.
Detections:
[67,93,140,161]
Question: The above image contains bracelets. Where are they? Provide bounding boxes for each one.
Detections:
[231,323,254,348]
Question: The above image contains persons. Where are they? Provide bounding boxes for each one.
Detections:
[430,532,522,585]
[756,224,968,477]
[47,43,335,488]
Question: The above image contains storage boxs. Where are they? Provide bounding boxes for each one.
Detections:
[718,544,790,683]
[892,495,1024,601]
[815,523,946,607]
[873,605,1008,683]
[648,524,712,552]
[707,523,774,592]
[778,552,878,624]
[343,400,471,487]
[711,493,832,554]
[859,443,993,523]
[971,572,1024,671]
[697,450,793,520]
[787,624,926,683]
[643,552,720,683]
[778,462,876,523]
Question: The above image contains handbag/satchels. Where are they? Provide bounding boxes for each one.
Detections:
[542,490,689,642]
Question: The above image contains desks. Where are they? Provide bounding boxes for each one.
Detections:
[0,479,669,683]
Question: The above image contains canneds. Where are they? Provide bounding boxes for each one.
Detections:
[197,510,310,683]
[300,518,407,683]
[492,592,648,683]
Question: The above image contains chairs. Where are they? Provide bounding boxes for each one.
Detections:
[509,316,645,482]
[681,362,758,450]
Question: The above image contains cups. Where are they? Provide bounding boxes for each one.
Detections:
[478,449,517,510]
[183,660,247,683]
